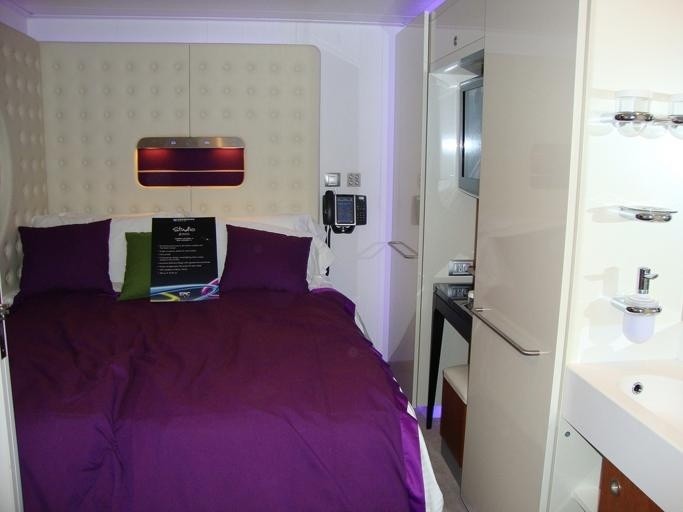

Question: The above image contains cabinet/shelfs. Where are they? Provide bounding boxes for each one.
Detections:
[431,1,484,67]
[460,2,595,511]
[388,11,474,409]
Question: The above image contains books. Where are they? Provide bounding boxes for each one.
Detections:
[147,216,221,302]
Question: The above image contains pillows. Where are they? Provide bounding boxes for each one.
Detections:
[17,217,116,297]
[221,223,313,295]
[119,231,152,298]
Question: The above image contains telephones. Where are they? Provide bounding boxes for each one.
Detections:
[323,190,367,233]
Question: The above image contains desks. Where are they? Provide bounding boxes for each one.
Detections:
[425,280,473,427]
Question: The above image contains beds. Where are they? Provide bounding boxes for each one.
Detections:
[12,210,442,512]
